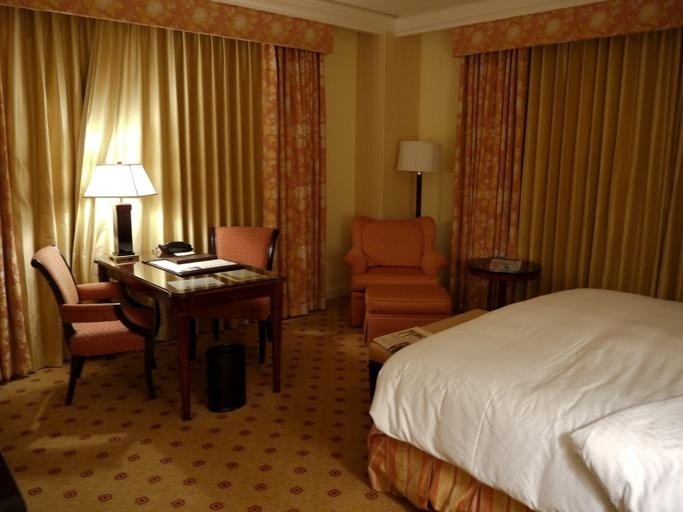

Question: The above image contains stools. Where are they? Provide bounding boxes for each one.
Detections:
[364,284,453,344]
[367,306,489,407]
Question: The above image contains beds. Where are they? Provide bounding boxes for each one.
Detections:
[366,288,683,512]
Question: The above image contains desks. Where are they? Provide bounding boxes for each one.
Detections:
[466,256,542,312]
[93,252,286,422]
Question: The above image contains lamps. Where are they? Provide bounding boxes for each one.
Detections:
[394,140,442,217]
[82,161,157,262]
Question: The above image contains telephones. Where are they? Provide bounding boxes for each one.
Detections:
[158,241,193,255]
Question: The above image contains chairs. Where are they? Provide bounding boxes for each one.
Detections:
[343,216,448,330]
[187,226,280,363]
[31,245,157,406]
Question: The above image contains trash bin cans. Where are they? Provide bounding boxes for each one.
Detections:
[206,343,246,413]
